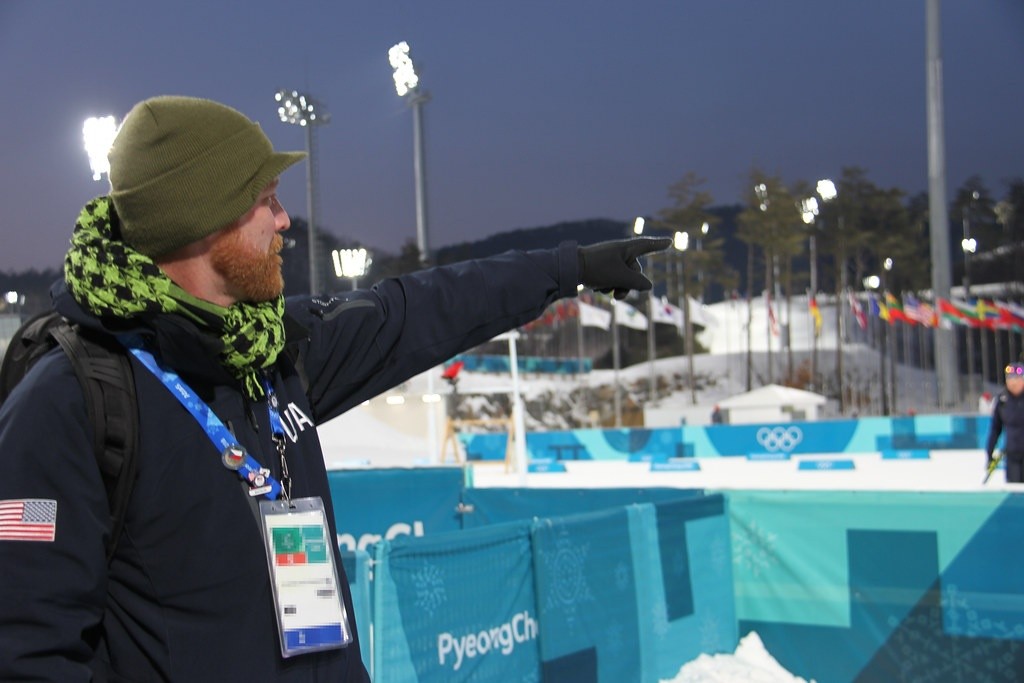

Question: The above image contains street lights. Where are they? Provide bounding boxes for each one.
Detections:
[800,197,823,395]
[960,189,983,414]
[753,183,774,384]
[634,216,645,235]
[275,89,333,296]
[388,41,432,267]
[863,275,880,348]
[332,241,373,291]
[674,232,697,405]
[81,115,117,181]
[816,179,847,415]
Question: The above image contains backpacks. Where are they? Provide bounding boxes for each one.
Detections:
[0,306,138,564]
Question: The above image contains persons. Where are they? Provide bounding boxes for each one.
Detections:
[985,363,1024,483]
[0,95,670,683]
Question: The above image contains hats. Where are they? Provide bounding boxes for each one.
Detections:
[106,95,309,263]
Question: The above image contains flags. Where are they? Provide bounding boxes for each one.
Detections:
[581,299,611,330]
[651,296,681,328]
[690,298,719,328]
[743,290,1024,332]
[615,300,647,331]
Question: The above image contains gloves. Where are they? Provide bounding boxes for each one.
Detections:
[578,236,672,299]
